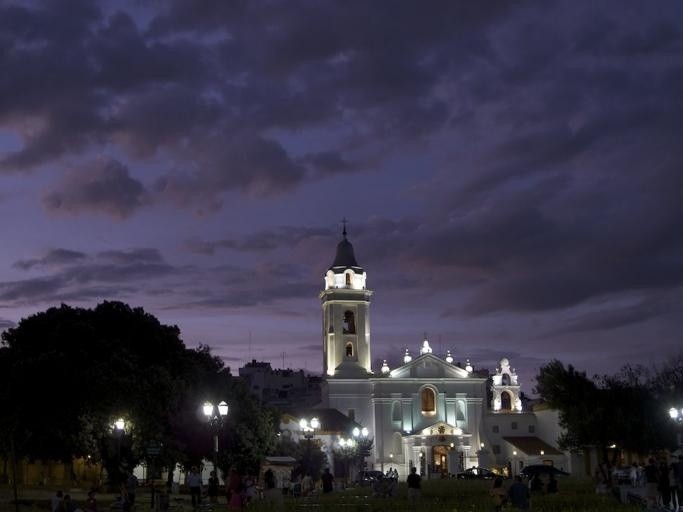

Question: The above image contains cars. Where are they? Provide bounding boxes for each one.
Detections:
[451,465,506,480]
[612,464,634,482]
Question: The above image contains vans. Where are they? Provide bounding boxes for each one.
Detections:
[281,473,303,494]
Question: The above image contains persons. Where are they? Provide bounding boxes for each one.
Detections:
[51,454,683,512]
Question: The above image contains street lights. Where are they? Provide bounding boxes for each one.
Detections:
[349,426,374,487]
[202,399,227,507]
[669,407,682,428]
[298,417,320,497]
[106,418,124,509]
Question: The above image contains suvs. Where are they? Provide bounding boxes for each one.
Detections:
[357,468,392,486]
[519,464,570,478]
